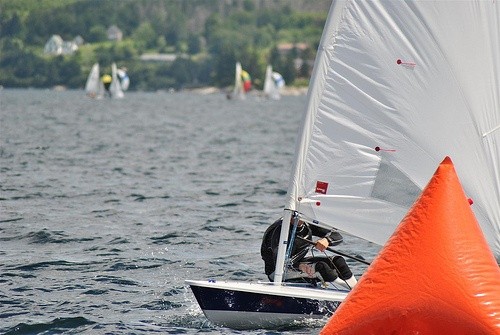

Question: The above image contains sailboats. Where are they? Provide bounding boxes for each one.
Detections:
[222,61,251,100]
[83,59,130,100]
[183,0,500,331]
[261,64,284,101]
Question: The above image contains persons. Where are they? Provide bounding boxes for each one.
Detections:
[261,214,357,291]
